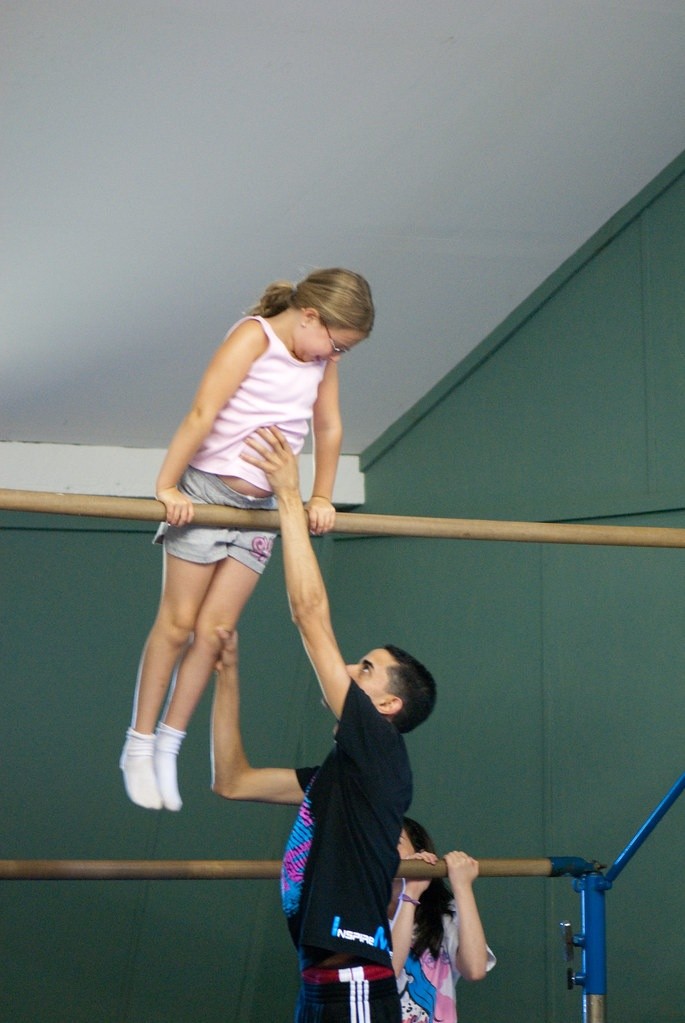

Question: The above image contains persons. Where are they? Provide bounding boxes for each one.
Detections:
[211,418,436,1023]
[117,266,377,813]
[388,815,498,1023]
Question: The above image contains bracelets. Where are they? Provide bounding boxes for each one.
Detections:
[396,891,420,908]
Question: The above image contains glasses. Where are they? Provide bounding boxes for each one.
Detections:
[319,316,350,355]
